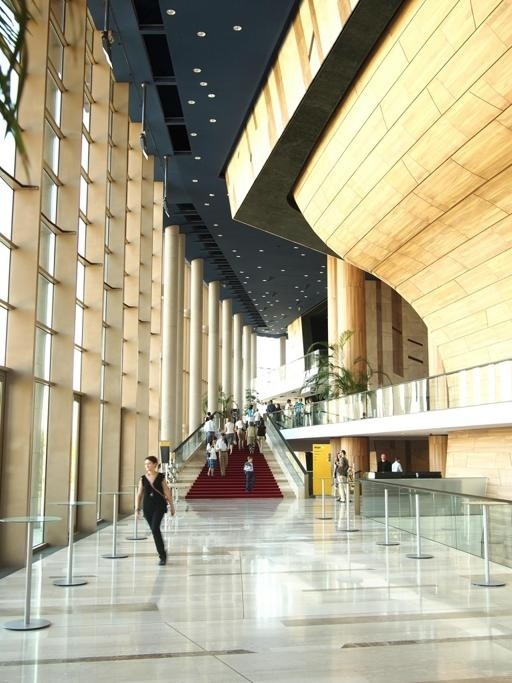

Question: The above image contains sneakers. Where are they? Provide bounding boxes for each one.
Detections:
[159,553,165,565]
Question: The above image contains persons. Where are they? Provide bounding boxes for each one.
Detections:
[204,398,314,478]
[333,450,351,502]
[392,457,404,472]
[135,456,175,565]
[243,456,255,491]
[378,452,392,472]
